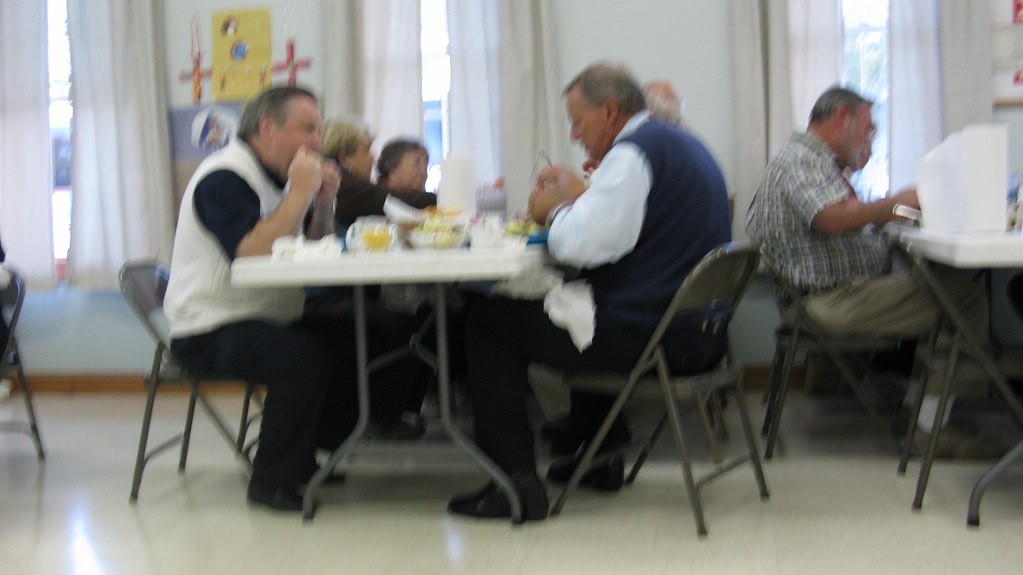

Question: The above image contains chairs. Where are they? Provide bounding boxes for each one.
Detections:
[0,263,44,461]
[117,257,266,505]
[900,234,1023,507]
[755,238,908,462]
[546,238,772,539]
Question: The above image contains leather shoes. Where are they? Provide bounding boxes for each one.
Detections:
[449,474,549,522]
[549,456,624,492]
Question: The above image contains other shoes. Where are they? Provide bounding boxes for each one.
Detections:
[421,400,442,415]
[541,418,635,451]
[916,427,1006,460]
[247,476,304,509]
[366,419,423,443]
[287,459,344,487]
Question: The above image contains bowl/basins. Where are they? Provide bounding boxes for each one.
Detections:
[409,227,465,250]
[345,222,399,252]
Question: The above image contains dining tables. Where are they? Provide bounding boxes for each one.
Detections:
[881,219,1023,524]
[225,226,551,528]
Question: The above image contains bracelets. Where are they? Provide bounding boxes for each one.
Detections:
[313,197,337,216]
[546,200,573,229]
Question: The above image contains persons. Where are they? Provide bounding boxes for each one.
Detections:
[747,88,1000,458]
[164,88,341,512]
[447,63,732,519]
[321,124,472,442]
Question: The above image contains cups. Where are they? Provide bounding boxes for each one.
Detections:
[476,188,508,221]
